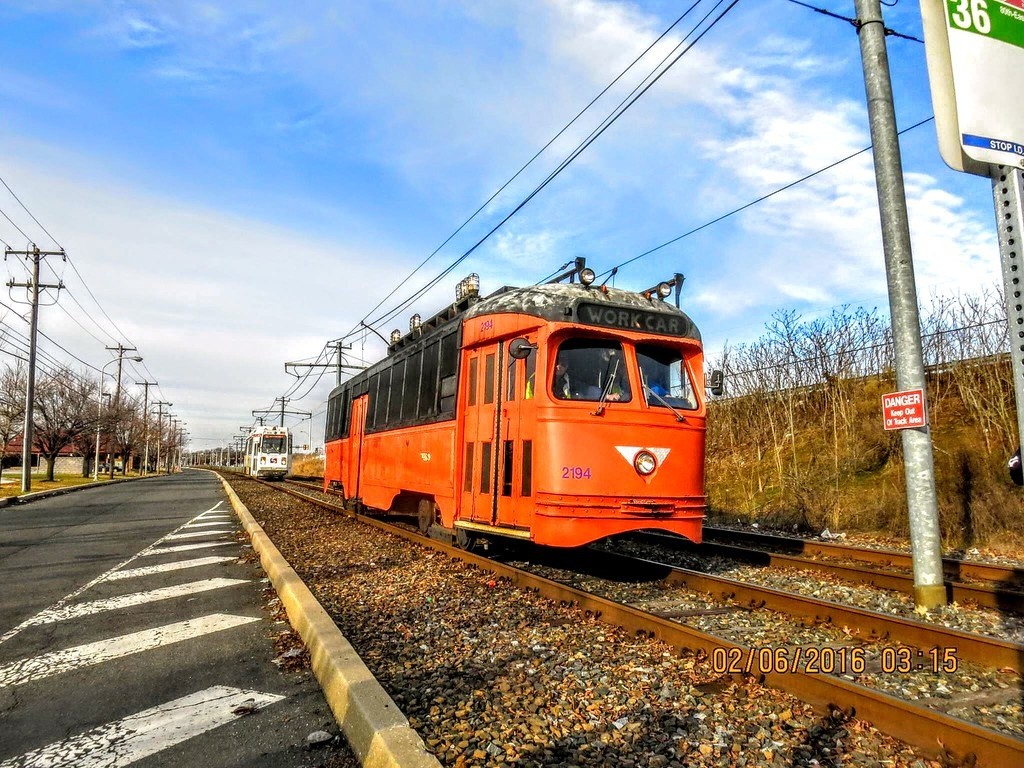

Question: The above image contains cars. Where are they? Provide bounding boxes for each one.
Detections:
[98,463,122,473]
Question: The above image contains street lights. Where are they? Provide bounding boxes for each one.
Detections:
[299,430,311,452]
[93,356,144,480]
[102,393,111,467]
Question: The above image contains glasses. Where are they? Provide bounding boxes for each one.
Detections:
[561,363,568,367]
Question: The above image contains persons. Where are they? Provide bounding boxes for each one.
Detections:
[598,358,672,405]
[524,349,580,402]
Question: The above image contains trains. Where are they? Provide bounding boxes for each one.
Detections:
[244,426,293,480]
[322,257,723,552]
